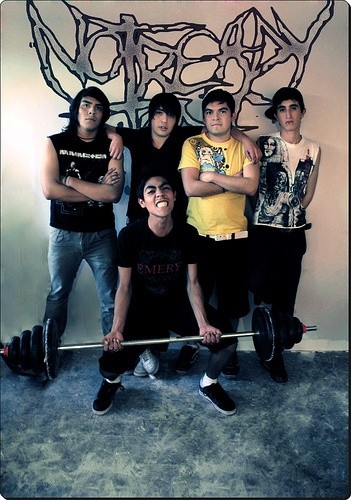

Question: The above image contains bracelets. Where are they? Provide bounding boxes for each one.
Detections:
[63,175,70,187]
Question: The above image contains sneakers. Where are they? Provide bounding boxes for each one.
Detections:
[199,382,236,416]
[221,352,240,378]
[176,346,200,373]
[92,380,125,414]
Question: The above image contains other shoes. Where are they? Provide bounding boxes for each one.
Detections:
[139,348,159,374]
[269,359,288,383]
[134,361,147,377]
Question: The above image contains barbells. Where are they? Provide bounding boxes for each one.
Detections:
[0,303,318,383]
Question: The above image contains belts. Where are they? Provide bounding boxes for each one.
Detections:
[199,231,248,242]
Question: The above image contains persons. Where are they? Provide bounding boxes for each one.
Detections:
[177,89,259,377]
[40,86,132,377]
[249,87,321,383]
[102,93,264,377]
[92,175,237,415]
